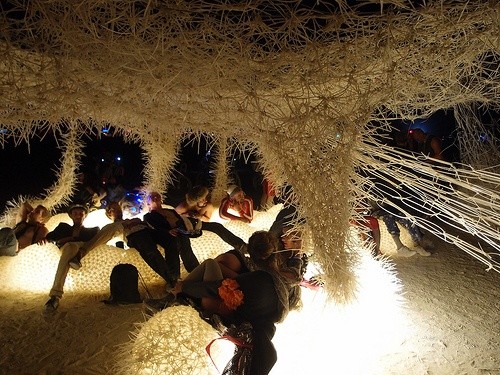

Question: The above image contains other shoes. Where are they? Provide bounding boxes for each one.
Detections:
[41,296,59,316]
[397,246,417,257]
[239,244,249,254]
[412,245,432,256]
[68,255,82,271]
[163,281,181,294]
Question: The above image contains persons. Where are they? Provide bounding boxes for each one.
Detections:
[65,151,308,374]
[0,202,49,256]
[361,125,444,258]
[44,204,124,316]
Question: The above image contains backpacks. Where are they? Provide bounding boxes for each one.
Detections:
[102,263,153,306]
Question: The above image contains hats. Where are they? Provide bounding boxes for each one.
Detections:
[67,197,88,220]
